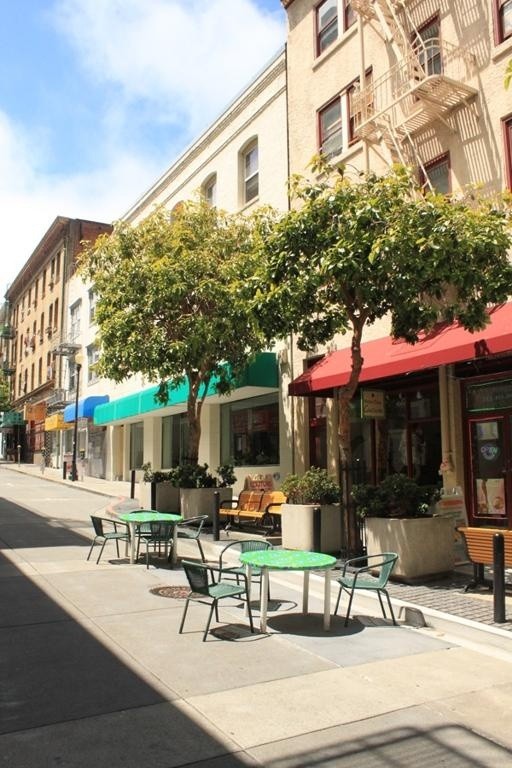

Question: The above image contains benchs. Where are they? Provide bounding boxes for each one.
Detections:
[452,525,512,594]
[218,489,289,538]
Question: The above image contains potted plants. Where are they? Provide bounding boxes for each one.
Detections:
[142,461,237,528]
[349,474,454,584]
[280,465,344,557]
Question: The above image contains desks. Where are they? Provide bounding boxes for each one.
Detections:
[118,513,183,564]
[238,550,337,634]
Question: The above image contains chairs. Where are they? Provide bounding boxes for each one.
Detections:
[126,510,159,557]
[218,540,274,603]
[179,559,254,642]
[333,550,398,629]
[136,519,175,570]
[88,514,130,565]
[164,514,209,563]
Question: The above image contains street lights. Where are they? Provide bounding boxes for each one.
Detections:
[68,364,82,481]
